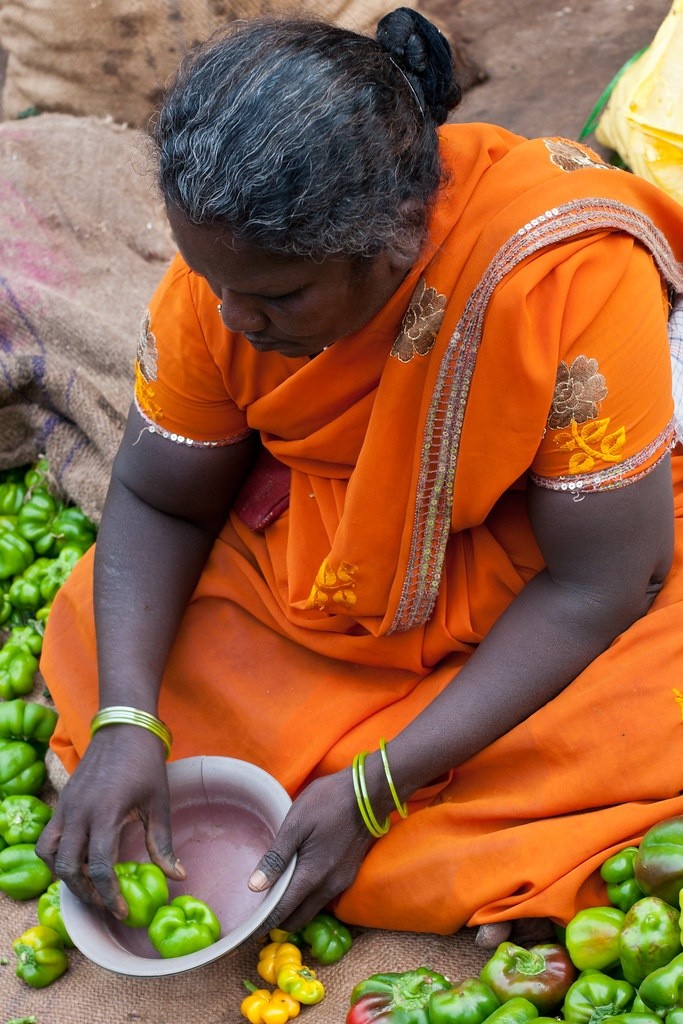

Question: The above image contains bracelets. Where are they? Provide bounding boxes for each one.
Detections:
[91,706,171,760]
[350,737,410,838]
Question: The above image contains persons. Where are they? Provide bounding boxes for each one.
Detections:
[40,8,682,949]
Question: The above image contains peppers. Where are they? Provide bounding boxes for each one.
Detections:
[0,451,222,989]
[239,812,683,1024]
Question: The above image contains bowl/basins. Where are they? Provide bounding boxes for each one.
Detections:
[60,755,298,977]
